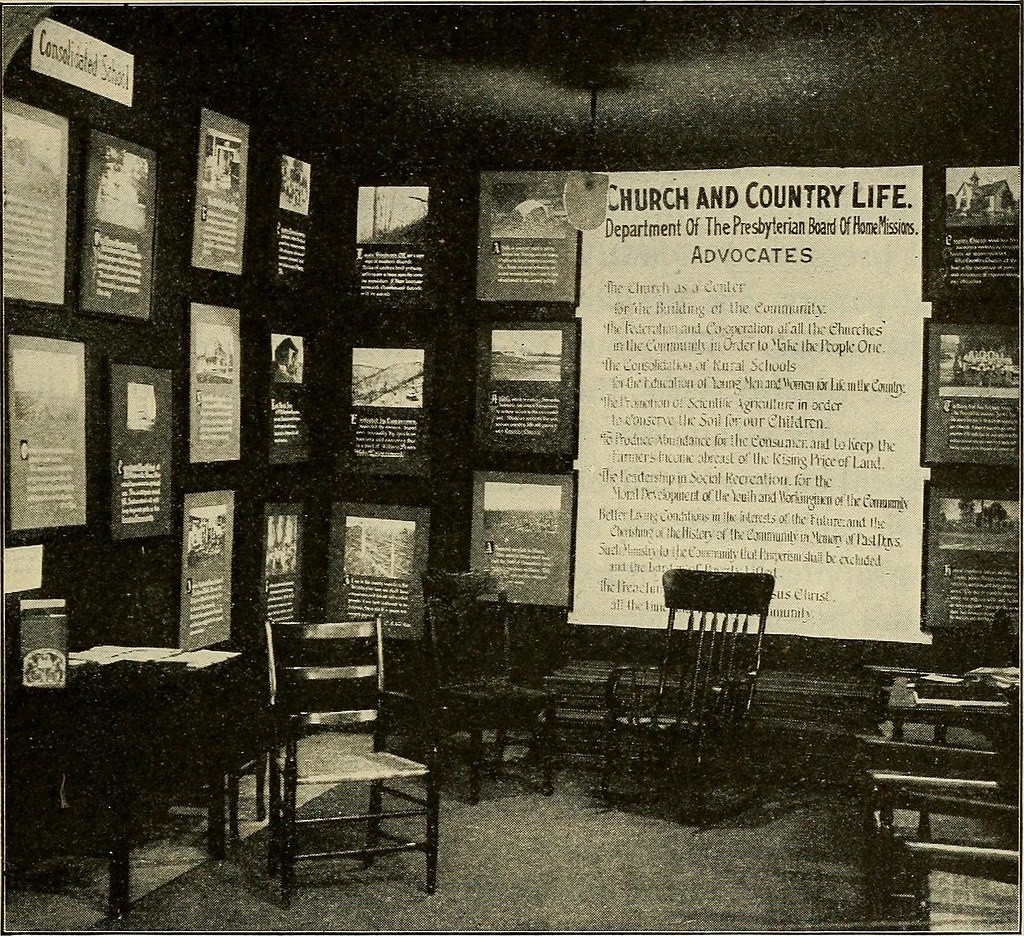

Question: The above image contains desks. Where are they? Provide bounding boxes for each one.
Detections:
[1,644,245,921]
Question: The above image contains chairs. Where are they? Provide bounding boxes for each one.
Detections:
[600,567,778,826]
[854,663,1019,932]
[264,616,441,910]
[419,567,558,804]
